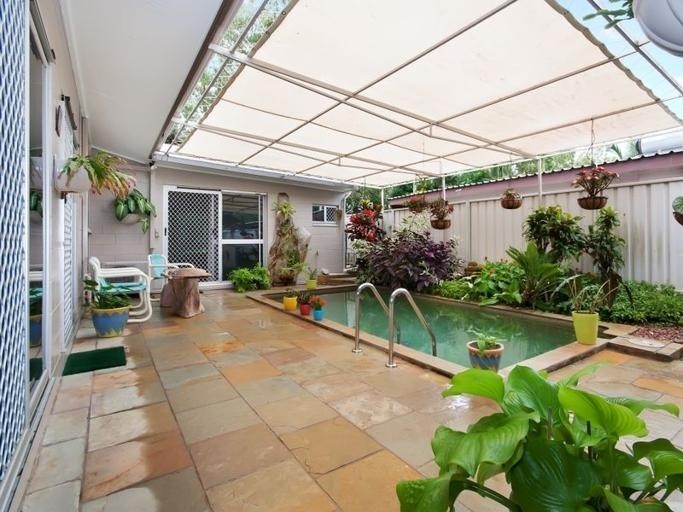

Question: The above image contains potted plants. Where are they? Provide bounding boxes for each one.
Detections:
[227,262,273,293]
[406,167,620,230]
[55,150,157,234]
[672,195,683,226]
[79,279,134,338]
[305,266,320,290]
[558,275,619,345]
[283,288,330,321]
[466,329,504,375]
[581,0,683,60]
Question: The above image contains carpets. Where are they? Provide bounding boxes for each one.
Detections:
[62,346,127,376]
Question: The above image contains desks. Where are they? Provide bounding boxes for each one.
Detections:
[160,268,212,319]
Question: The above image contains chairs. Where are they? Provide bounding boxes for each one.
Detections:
[88,253,195,323]
